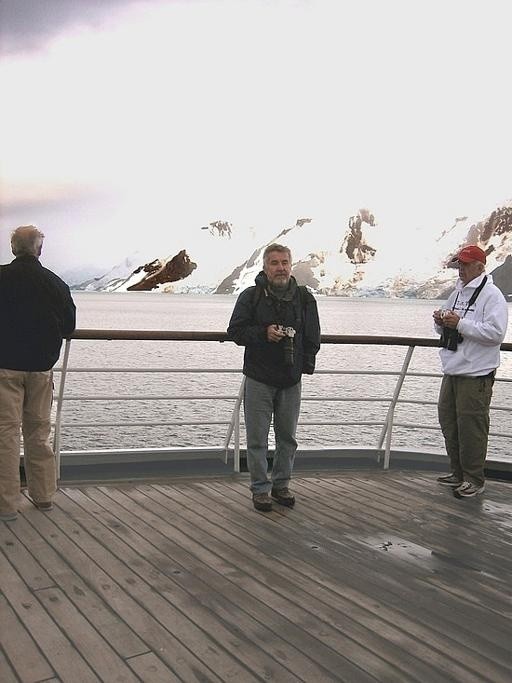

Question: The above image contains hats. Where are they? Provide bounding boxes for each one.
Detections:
[452,245,487,265]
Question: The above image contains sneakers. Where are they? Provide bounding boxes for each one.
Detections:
[253,492,272,512]
[0,514,16,521]
[436,475,460,486]
[271,486,296,506]
[455,480,485,498]
[28,488,53,511]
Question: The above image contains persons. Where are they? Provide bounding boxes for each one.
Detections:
[0,223,75,521]
[433,246,508,498]
[226,242,320,510]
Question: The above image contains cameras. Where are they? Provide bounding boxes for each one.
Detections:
[439,309,451,320]
[276,327,296,338]
